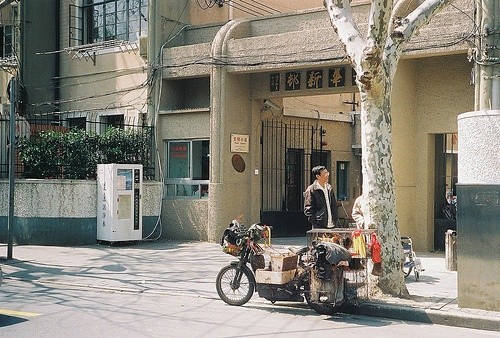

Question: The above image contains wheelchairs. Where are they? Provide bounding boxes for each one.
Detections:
[401,237,425,282]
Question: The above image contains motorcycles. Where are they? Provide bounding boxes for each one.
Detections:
[216,217,364,316]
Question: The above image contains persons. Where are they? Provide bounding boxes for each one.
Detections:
[304,166,340,246]
[352,192,384,277]
[194,186,208,196]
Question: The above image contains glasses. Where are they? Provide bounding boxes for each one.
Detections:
[323,172,330,177]
